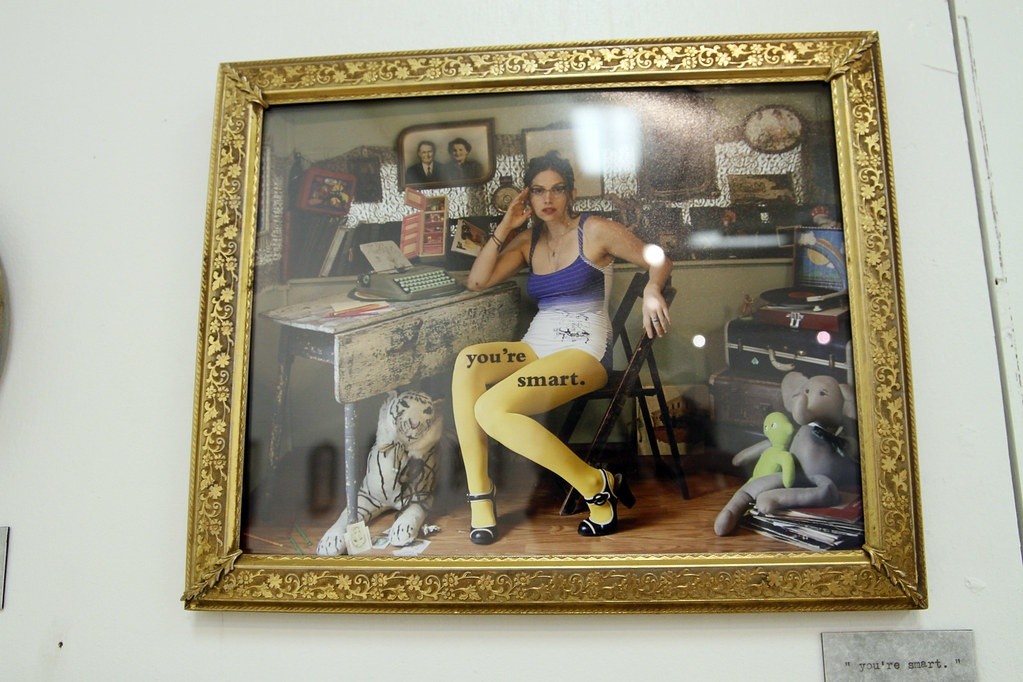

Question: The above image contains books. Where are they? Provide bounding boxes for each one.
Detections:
[739,485,867,551]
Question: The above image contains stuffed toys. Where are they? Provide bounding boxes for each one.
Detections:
[712,372,857,536]
[313,388,464,557]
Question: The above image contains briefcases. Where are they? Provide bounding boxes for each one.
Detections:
[724,314,853,384]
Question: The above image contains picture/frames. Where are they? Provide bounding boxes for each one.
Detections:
[181,32,928,612]
[348,158,383,204]
[397,117,495,193]
[520,121,605,200]
[741,104,808,154]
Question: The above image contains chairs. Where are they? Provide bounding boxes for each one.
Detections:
[532,266,693,516]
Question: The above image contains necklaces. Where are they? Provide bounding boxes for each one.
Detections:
[546,221,569,256]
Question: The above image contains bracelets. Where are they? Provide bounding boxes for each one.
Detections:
[492,235,504,246]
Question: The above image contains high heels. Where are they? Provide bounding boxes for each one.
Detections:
[578,469,635,537]
[466,477,498,545]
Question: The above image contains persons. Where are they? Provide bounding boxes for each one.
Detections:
[452,151,673,542]
[445,137,482,179]
[406,141,448,181]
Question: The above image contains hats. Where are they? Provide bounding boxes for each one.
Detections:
[448,137,470,153]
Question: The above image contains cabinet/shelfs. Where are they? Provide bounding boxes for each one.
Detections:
[277,259,794,447]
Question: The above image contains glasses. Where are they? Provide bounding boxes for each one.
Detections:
[529,186,565,197]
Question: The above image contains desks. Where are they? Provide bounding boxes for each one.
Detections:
[257,277,521,530]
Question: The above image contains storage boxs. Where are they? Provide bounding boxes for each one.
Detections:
[724,314,854,388]
[708,369,794,429]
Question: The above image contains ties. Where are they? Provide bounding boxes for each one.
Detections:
[426,166,432,177]
